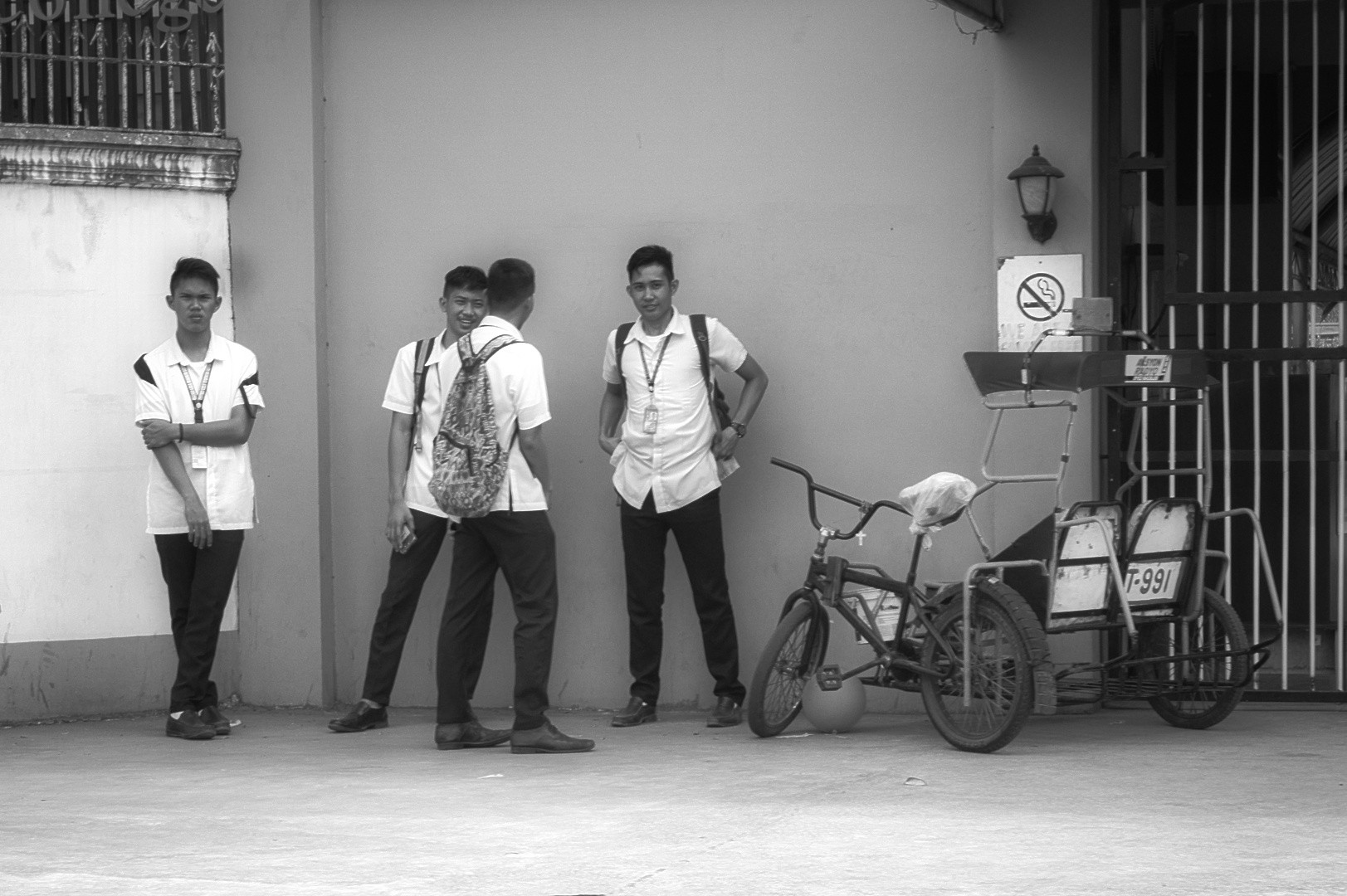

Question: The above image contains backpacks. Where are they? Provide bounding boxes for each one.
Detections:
[428,329,529,517]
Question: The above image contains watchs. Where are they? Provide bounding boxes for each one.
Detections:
[729,422,747,439]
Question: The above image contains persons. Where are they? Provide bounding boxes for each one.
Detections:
[434,259,595,754]
[328,265,488,733]
[598,244,770,728]
[133,259,265,739]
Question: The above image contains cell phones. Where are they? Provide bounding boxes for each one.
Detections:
[399,523,417,555]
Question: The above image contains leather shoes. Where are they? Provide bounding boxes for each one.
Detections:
[435,720,511,749]
[200,706,230,733]
[165,711,215,738]
[328,702,388,730]
[611,695,656,726]
[511,722,594,753]
[706,696,742,726]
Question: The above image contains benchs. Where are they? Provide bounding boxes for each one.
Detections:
[974,497,1202,627]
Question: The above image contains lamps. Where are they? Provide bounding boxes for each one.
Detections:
[1007,145,1066,243]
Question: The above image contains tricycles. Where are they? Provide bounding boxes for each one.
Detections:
[748,299,1284,754]
